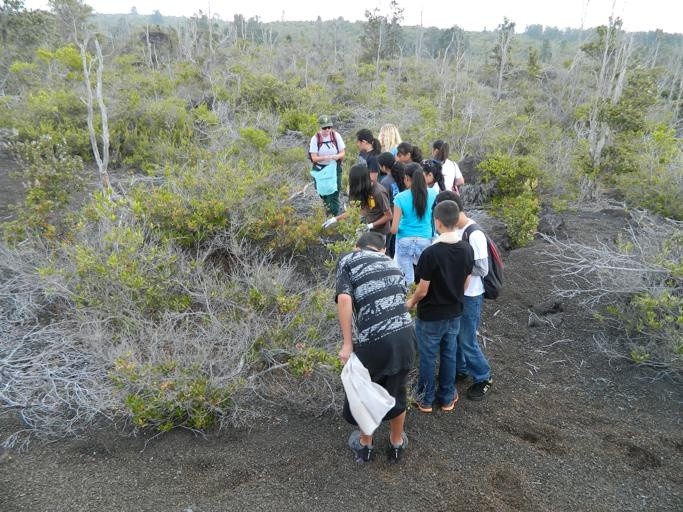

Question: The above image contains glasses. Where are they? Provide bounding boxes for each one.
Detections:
[321,126,332,130]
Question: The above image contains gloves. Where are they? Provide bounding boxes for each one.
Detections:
[319,216,337,228]
[355,222,373,234]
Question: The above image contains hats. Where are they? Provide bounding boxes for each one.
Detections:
[318,115,333,128]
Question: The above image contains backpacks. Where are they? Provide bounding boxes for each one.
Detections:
[462,223,505,300]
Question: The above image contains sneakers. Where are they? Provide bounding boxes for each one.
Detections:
[441,392,459,412]
[348,429,375,465]
[387,430,409,464]
[413,390,433,413]
[467,376,494,402]
[453,371,467,383]
